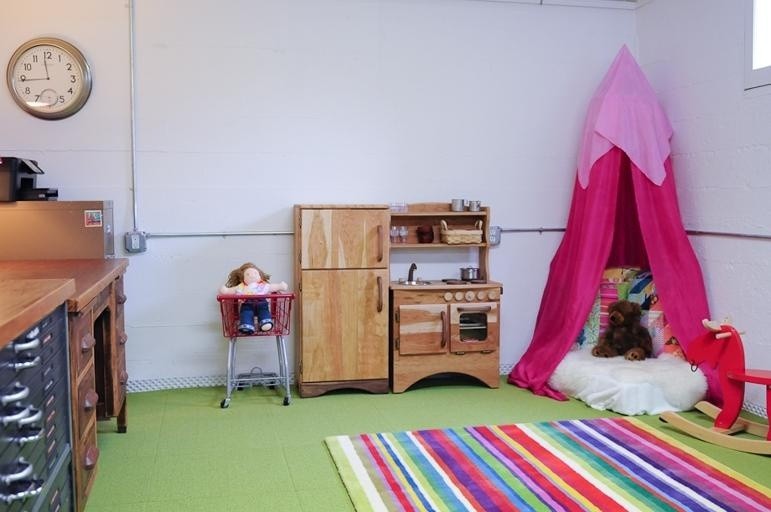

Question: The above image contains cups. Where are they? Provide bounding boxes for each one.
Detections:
[469,200,481,212]
[389,199,407,214]
[450,198,468,212]
[390,225,408,244]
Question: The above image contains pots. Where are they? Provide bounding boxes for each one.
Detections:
[459,267,480,281]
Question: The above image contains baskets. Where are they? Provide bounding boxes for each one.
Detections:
[440,219,484,245]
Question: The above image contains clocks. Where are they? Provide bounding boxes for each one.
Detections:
[6,34,93,122]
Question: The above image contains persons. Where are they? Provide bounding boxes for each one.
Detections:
[218,261,289,334]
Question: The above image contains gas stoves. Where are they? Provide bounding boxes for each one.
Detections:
[441,277,502,302]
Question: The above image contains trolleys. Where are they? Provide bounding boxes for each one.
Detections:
[216,289,298,408]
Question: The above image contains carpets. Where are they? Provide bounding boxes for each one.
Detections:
[321,416,771,510]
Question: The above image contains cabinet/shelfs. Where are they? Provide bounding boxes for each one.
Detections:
[390,200,501,391]
[0,278,82,512]
[0,200,115,261]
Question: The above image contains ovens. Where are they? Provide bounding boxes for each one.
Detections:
[447,302,499,355]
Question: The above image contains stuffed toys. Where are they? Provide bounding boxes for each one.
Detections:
[626,271,658,309]
[592,300,652,361]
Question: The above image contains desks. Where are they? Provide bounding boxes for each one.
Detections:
[0,260,133,512]
